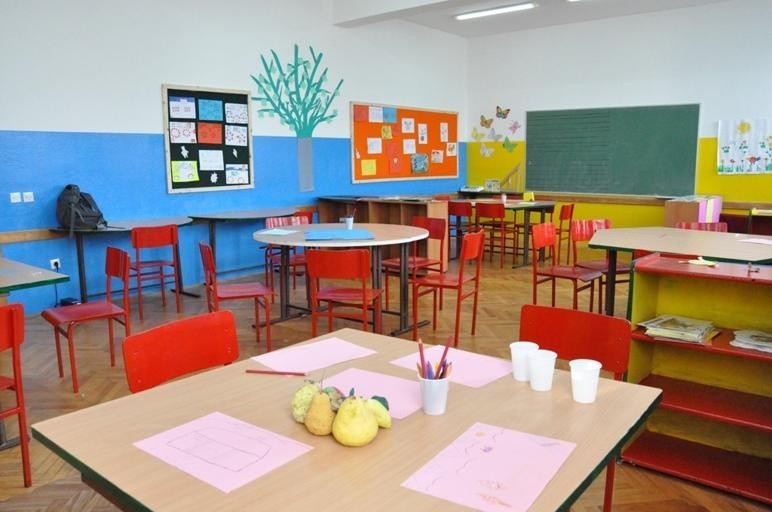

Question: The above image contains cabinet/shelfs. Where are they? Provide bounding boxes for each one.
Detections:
[616,251,772,501]
[315,195,450,275]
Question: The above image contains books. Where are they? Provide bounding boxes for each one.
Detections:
[637,313,722,347]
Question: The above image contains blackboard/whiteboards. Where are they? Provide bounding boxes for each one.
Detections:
[162,85,255,195]
[524,103,700,196]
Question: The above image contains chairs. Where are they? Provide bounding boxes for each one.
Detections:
[530,218,772,315]
[449,198,575,268]
[302,249,384,338]
[266,215,310,304]
[124,311,237,393]
[198,242,276,352]
[129,223,181,320]
[40,246,130,392]
[382,218,446,312]
[411,228,485,347]
[0,303,32,486]
[518,303,631,511]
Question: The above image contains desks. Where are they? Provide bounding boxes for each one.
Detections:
[0,253,70,452]
[252,221,431,337]
[48,217,200,303]
[189,207,298,287]
[28,322,666,512]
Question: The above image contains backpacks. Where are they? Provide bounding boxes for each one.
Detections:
[56,184,126,240]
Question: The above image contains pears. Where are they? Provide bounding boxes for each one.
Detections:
[292,383,318,424]
[305,391,336,435]
[367,398,392,429]
[330,396,379,446]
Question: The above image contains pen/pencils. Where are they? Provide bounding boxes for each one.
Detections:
[413,337,455,380]
[246,370,306,376]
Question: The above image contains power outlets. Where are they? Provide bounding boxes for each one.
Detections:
[50,259,60,272]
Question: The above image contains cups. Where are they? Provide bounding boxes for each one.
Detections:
[509,341,539,382]
[569,359,602,403]
[529,349,558,392]
[502,196,507,204]
[418,368,449,415]
[346,217,354,230]
